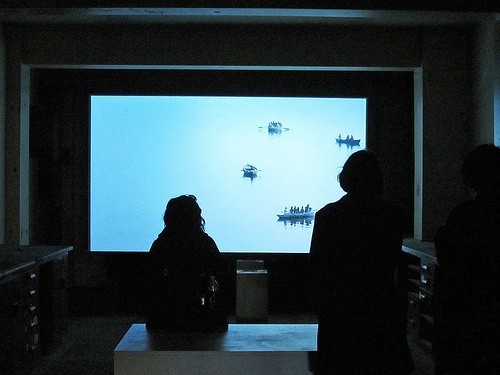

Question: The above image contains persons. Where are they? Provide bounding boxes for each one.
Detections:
[134,195,229,332]
[433,143,500,375]
[307,150,414,375]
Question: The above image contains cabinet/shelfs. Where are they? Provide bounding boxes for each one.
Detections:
[0,244,74,375]
[403,239,438,342]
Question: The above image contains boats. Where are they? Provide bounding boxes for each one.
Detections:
[268,122,282,134]
[335,139,361,144]
[277,212,316,219]
[243,163,258,177]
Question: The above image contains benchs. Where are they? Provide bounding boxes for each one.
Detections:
[113,324,320,375]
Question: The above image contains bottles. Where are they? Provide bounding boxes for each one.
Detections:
[207,276,219,311]
